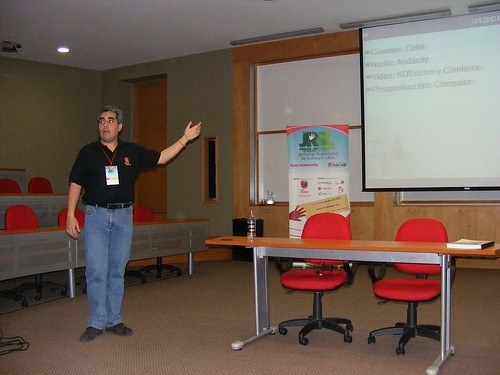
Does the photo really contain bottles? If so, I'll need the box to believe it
[247,210,256,240]
[267,191,275,204]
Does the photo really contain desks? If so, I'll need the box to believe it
[0,168,26,192]
[205,236,500,375]
[0,193,85,229]
[0,218,209,298]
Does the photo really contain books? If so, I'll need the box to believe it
[447,239,494,250]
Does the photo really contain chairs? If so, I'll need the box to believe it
[275,213,359,345]
[28,177,52,193]
[58,208,147,293]
[0,179,21,192]
[6,206,67,300]
[134,207,182,279]
[368,218,456,355]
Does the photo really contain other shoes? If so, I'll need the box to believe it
[79,326,103,342]
[105,323,133,336]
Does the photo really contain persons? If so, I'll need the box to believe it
[65,104,202,344]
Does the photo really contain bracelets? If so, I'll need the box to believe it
[178,138,187,147]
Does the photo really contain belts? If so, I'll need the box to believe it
[87,201,132,209]
